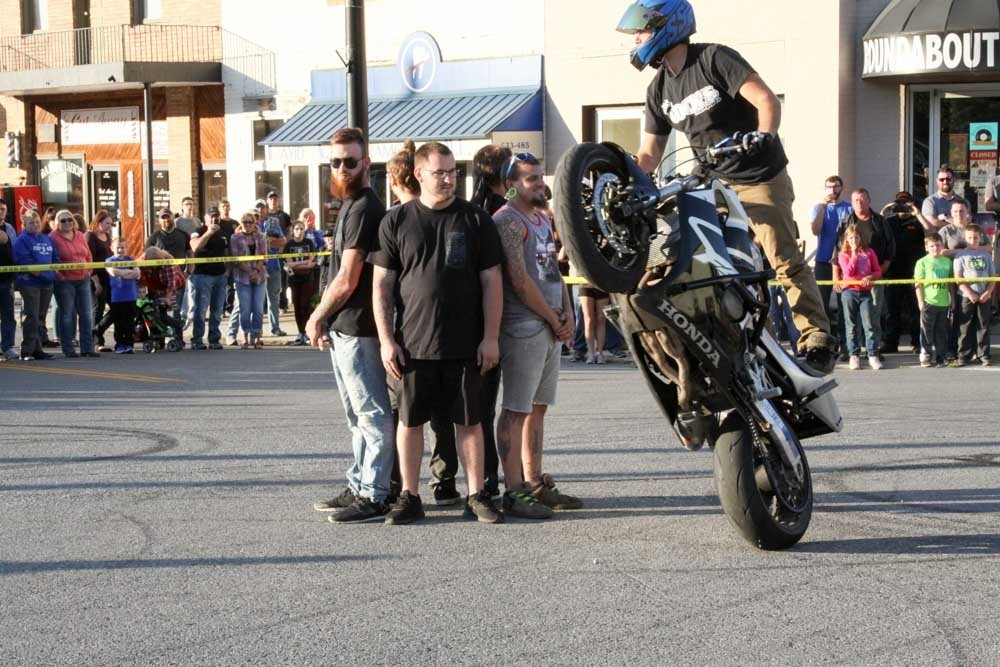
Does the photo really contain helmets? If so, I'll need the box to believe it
[615,0,697,71]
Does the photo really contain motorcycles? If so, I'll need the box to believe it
[552,130,843,551]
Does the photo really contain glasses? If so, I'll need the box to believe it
[60,218,73,223]
[424,168,458,179]
[331,156,365,170]
[242,220,254,225]
[939,176,954,182]
[506,152,535,177]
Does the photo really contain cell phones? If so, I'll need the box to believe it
[214,218,220,225]
[892,204,909,212]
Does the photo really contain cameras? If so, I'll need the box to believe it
[48,207,56,214]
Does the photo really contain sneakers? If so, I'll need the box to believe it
[868,356,883,369]
[313,472,587,525]
[849,356,861,370]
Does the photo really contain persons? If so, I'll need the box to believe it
[302,129,579,527]
[0,191,329,362]
[539,164,999,373]
[614,0,843,375]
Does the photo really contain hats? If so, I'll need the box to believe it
[207,206,220,217]
[255,199,268,208]
[158,207,173,220]
[267,192,280,198]
[250,209,260,216]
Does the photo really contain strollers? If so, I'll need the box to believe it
[131,279,187,354]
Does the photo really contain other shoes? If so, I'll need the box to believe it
[921,355,993,369]
[114,346,126,354]
[125,345,133,354]
[802,347,838,375]
[1,337,101,359]
[191,328,305,350]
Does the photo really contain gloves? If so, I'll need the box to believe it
[734,132,773,155]
